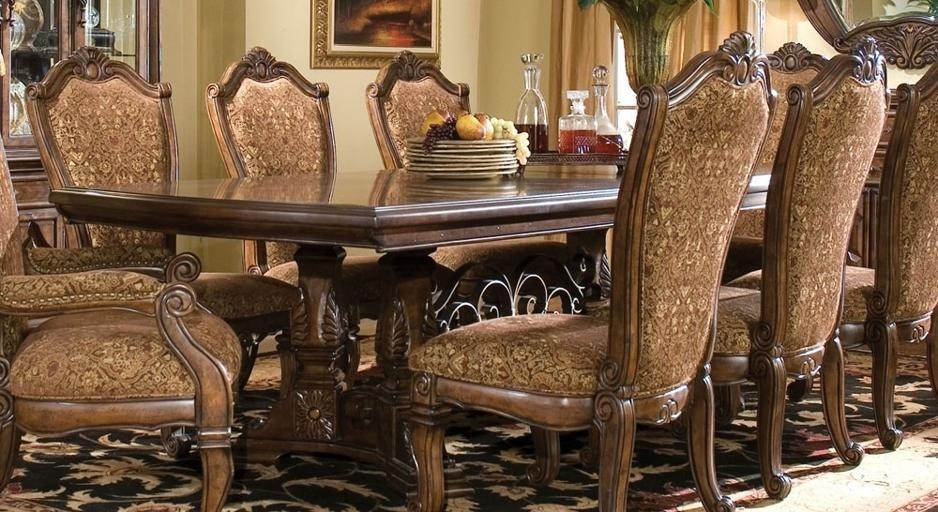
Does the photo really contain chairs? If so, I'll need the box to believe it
[363,51,585,341]
[714,59,938,452]
[577,35,893,498]
[402,29,779,510]
[0,64,242,510]
[719,41,832,284]
[206,47,465,419]
[27,46,304,454]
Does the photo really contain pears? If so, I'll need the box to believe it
[456,115,486,140]
[422,112,445,135]
[474,113,493,139]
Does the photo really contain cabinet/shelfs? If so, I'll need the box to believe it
[846,104,899,270]
[0,0,159,249]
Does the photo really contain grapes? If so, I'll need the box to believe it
[422,118,458,151]
[492,118,530,165]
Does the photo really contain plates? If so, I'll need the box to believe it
[405,133,519,180]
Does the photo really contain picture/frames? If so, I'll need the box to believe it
[309,0,443,69]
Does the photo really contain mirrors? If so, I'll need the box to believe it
[798,0,938,69]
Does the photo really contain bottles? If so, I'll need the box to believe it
[508,52,626,155]
